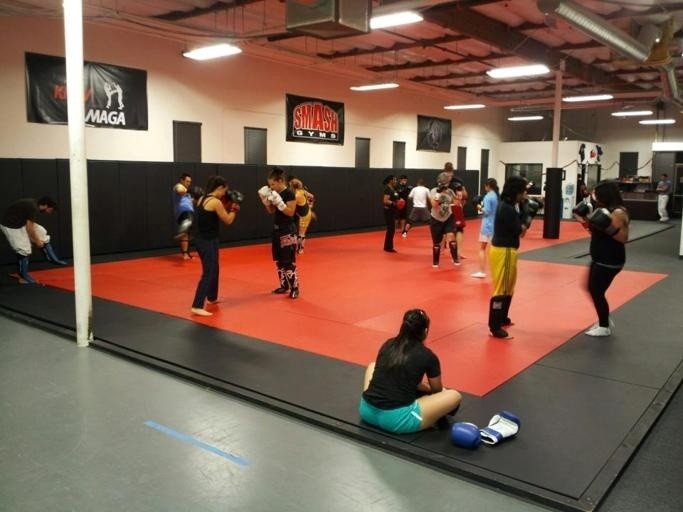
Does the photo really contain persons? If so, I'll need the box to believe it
[0,196,67,285]
[580,184,591,204]
[104,80,125,110]
[359,308,462,434]
[289,179,318,254]
[656,173,672,222]
[191,175,240,316]
[573,180,629,337]
[487,175,543,338]
[382,162,469,267]
[471,178,499,278]
[258,167,299,299]
[171,174,199,260]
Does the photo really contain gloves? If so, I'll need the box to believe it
[192,186,203,200]
[471,195,482,209]
[573,200,593,229]
[588,207,620,238]
[257,185,288,213]
[221,190,245,214]
[518,198,546,229]
[391,198,405,209]
[480,410,521,446]
[450,422,482,451]
